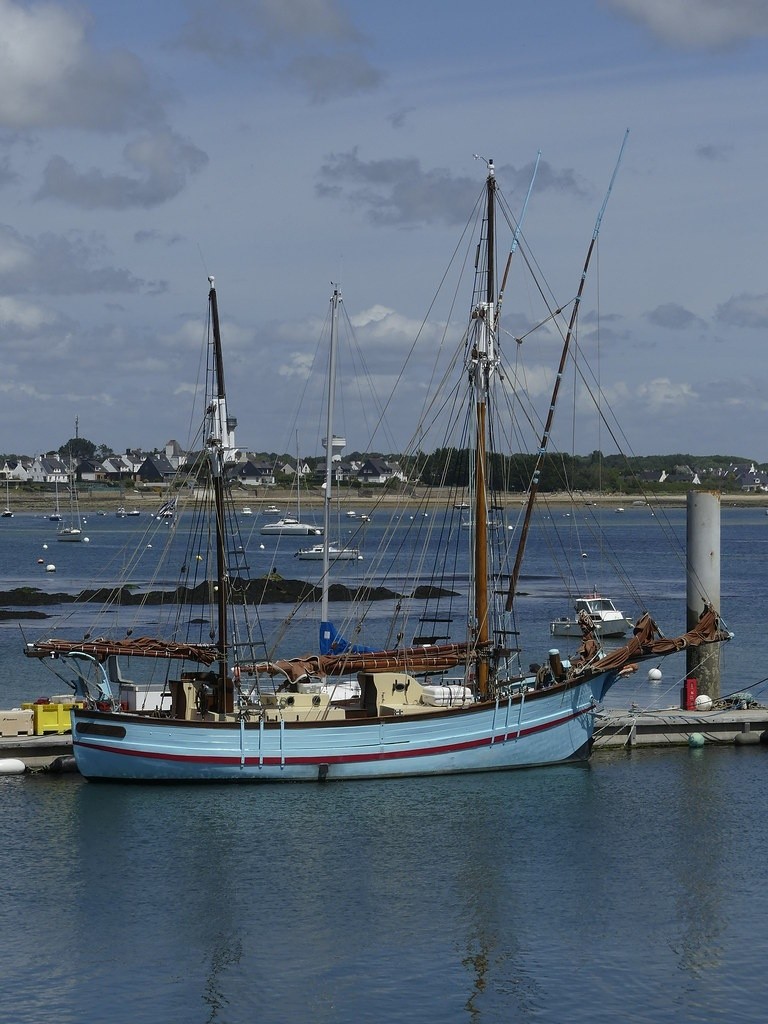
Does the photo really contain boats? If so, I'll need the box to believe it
[300,542,362,562]
[548,597,634,637]
[262,505,281,514]
[261,517,324,536]
[240,507,254,518]
[116,508,141,518]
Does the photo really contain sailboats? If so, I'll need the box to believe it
[55,444,85,541]
[49,475,63,521]
[1,475,14,517]
[51,150,735,782]
